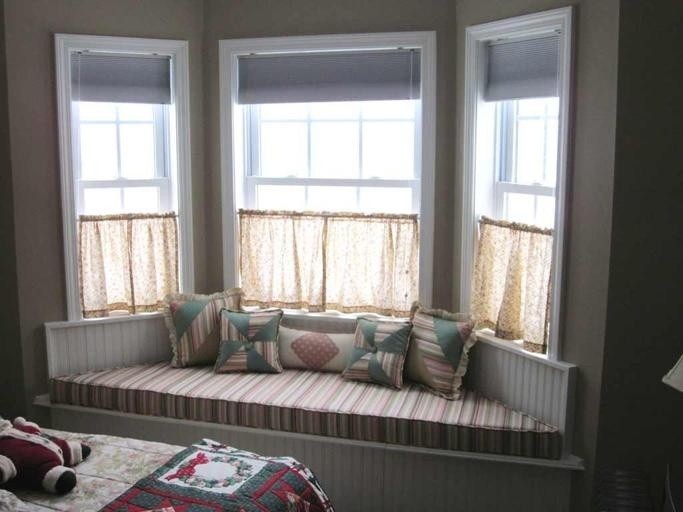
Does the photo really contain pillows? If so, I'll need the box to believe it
[403,301,480,400]
[342,318,414,391]
[278,323,354,372]
[162,288,245,368]
[214,308,284,373]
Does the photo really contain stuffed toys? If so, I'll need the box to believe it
[0,417,91,496]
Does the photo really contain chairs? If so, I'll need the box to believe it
[589,464,677,512]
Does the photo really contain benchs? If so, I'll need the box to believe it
[44,311,578,512]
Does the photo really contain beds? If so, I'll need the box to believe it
[0,417,334,512]
